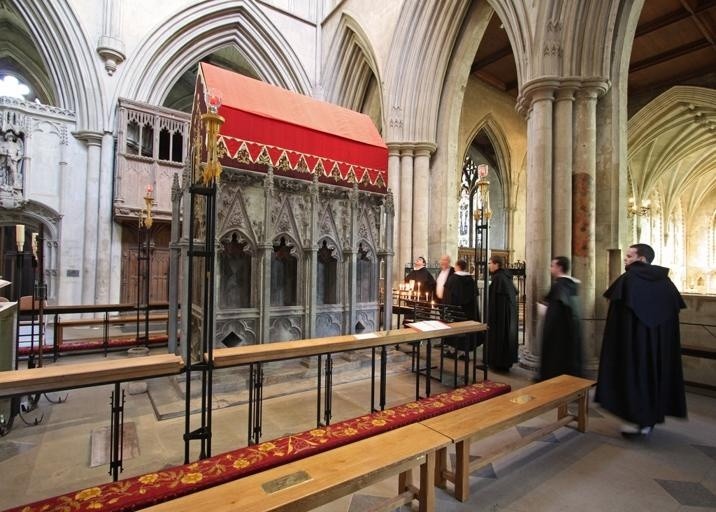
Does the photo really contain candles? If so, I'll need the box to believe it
[16,224,25,243]
[399,281,429,302]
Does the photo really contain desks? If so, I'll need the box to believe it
[18,301,180,364]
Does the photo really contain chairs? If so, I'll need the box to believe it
[19,296,48,347]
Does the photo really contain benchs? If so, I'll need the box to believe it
[419,374,600,498]
[137,424,454,510]
[4,380,515,511]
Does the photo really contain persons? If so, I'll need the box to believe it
[434,252,457,351]
[442,259,485,363]
[403,255,437,348]
[531,254,587,379]
[475,254,519,375]
[593,242,689,437]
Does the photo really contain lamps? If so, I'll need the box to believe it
[627,196,652,218]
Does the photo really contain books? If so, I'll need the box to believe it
[405,317,452,332]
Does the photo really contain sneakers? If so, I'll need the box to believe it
[619,423,655,437]
[434,343,470,362]
